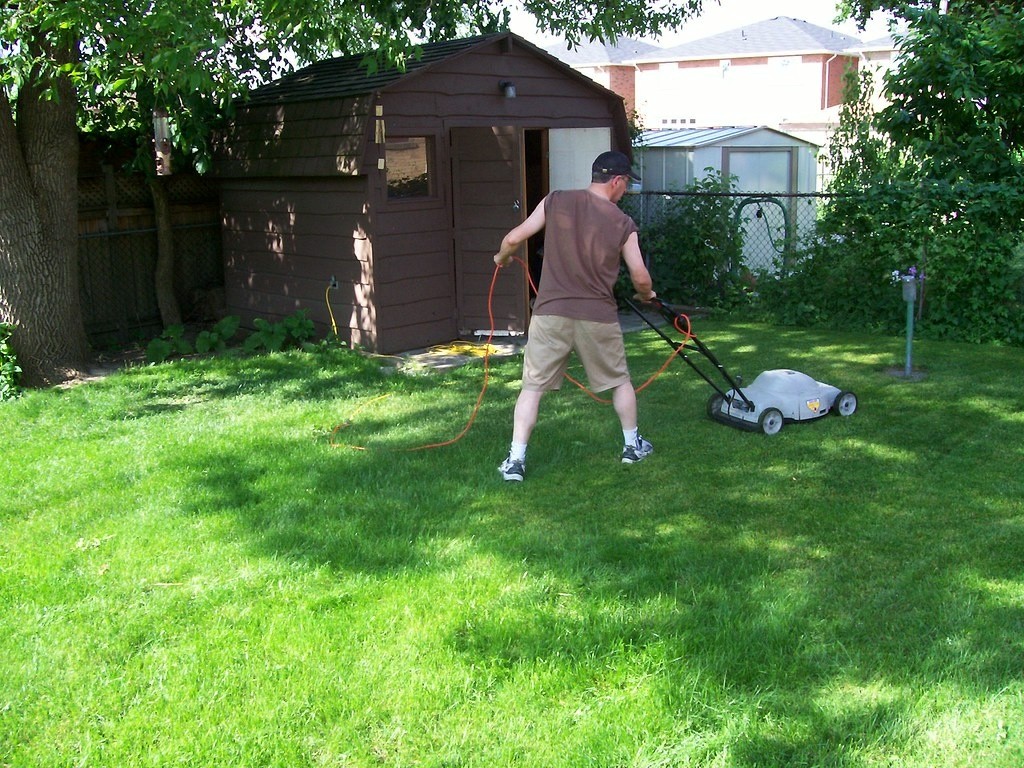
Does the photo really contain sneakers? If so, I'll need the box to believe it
[499,450,526,483]
[622,435,653,464]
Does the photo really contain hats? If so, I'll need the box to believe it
[592,151,641,181]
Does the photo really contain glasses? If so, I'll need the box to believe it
[613,174,630,189]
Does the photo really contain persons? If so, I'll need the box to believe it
[493,151,657,481]
